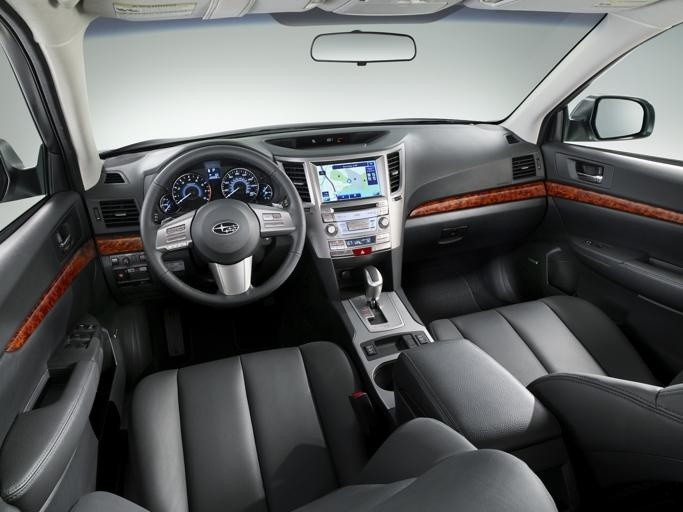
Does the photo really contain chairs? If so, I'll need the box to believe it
[428,295,683,489]
[70,342,559,512]
